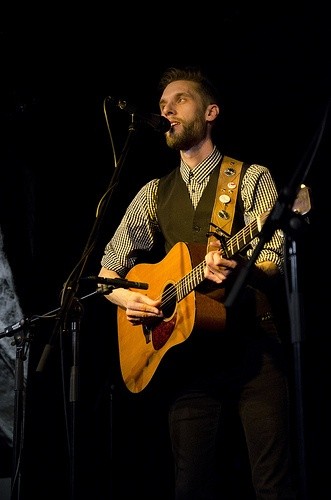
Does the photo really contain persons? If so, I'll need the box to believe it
[96,66,291,500]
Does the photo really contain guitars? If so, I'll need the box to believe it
[117,181,316,396]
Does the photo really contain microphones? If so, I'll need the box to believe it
[107,96,171,132]
[85,275,148,290]
